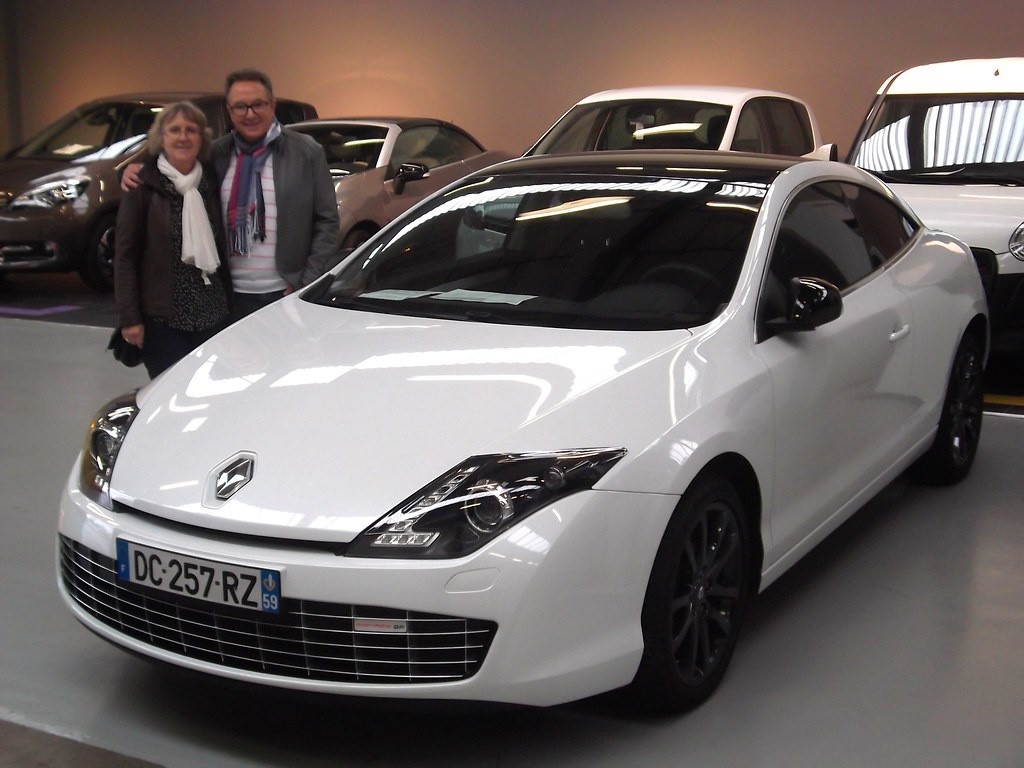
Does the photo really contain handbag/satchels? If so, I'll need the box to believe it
[107,327,144,367]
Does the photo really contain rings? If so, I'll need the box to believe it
[125,337,128,341]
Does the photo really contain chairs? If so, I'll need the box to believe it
[132,113,155,136]
[543,186,651,294]
[328,136,359,163]
[625,104,709,149]
[422,139,452,162]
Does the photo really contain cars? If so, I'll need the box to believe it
[0,91,321,291]
[55,148,992,717]
[284,116,520,254]
[453,85,838,272]
[843,56,1024,276]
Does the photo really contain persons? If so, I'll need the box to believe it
[120,69,341,323]
[115,101,236,383]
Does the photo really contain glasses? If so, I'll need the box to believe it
[162,128,200,137]
[227,100,271,116]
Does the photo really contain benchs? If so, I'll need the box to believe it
[705,116,805,155]
